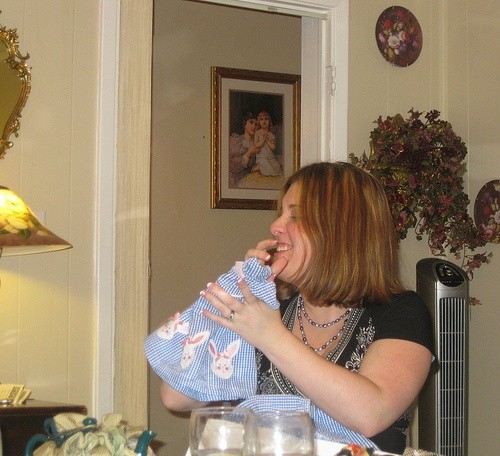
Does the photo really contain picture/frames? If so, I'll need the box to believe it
[210,66,301,212]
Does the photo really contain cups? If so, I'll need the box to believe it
[244,410,316,456]
[189,406,260,456]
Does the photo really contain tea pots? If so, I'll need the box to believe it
[25,417,158,455]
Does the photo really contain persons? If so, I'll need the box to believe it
[160,162,434,456]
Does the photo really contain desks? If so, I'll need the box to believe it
[0,399,88,456]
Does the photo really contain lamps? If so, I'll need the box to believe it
[0,186,73,256]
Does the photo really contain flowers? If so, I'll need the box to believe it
[348,108,498,307]
[473,180,500,243]
[375,6,422,69]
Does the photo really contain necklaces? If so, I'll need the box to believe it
[297,293,352,352]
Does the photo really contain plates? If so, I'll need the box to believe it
[375,6,423,67]
[474,180,500,244]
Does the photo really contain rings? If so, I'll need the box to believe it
[225,309,235,323]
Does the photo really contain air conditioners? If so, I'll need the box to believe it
[416,257,469,456]
